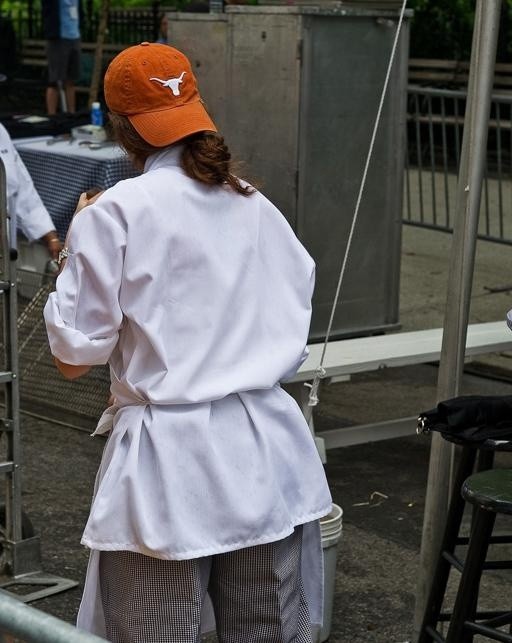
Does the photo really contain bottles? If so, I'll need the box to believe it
[91,102,104,127]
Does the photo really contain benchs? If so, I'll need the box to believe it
[7,36,130,94]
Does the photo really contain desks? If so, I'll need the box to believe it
[10,136,142,248]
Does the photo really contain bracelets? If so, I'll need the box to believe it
[48,237,60,242]
[56,246,68,263]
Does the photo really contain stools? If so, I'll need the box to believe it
[416,424,512,643]
[444,468,512,643]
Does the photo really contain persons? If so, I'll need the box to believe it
[37,0,87,115]
[40,41,334,643]
[1,120,65,265]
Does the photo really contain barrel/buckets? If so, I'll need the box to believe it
[317,502,345,643]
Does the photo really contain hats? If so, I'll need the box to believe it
[104,42,217,147]
